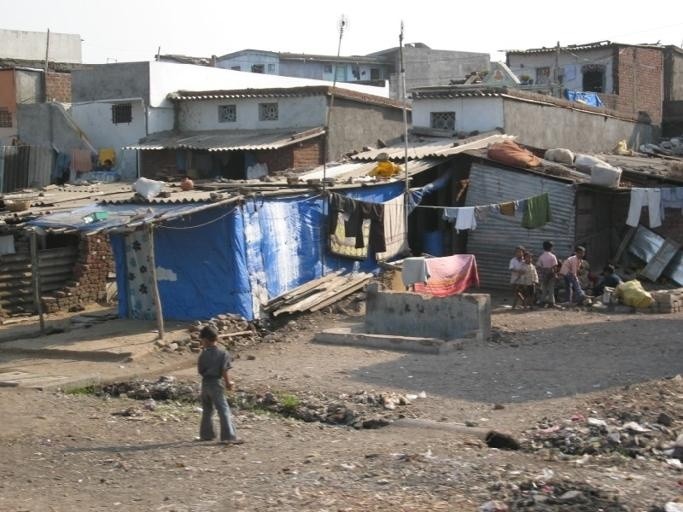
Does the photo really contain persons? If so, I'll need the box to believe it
[511,239,620,308]
[198,327,245,444]
